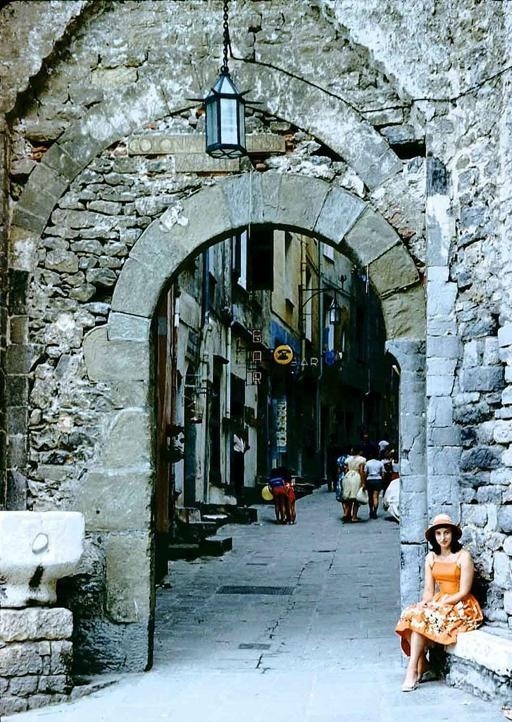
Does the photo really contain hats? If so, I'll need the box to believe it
[425,511,464,541]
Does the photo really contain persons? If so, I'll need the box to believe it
[269,467,298,526]
[394,525,483,692]
[334,448,400,522]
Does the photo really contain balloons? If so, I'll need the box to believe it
[262,485,273,500]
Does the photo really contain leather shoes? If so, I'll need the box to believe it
[400,673,419,692]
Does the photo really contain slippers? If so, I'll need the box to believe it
[341,512,363,522]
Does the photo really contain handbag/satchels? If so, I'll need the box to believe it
[271,486,286,496]
[356,485,369,505]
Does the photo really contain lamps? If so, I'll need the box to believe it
[184,0,262,162]
[298,284,344,328]
